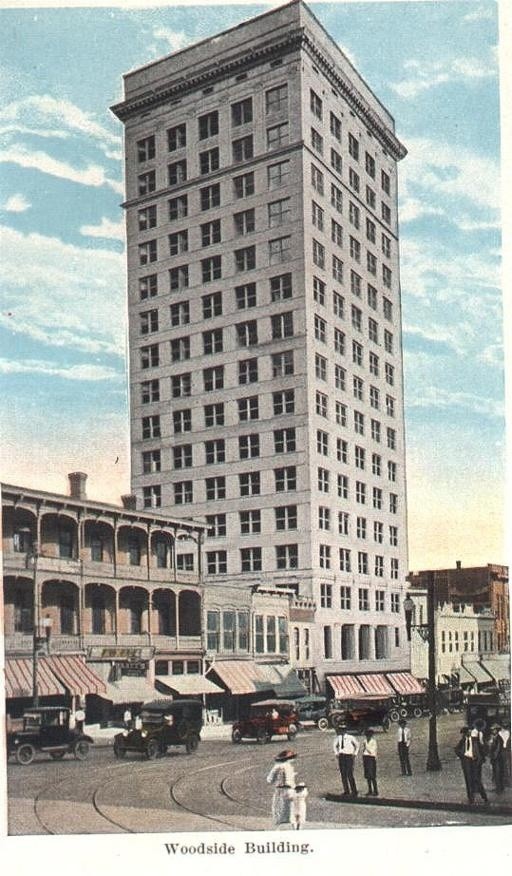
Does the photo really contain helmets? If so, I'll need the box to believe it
[473,718,487,731]
[459,724,472,735]
[490,723,500,731]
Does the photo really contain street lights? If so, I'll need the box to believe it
[29,611,54,708]
[400,570,444,770]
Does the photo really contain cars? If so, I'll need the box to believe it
[113,698,206,759]
[462,677,511,746]
[228,690,436,744]
[7,705,96,765]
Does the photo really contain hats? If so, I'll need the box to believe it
[273,750,298,761]
[294,782,307,789]
[337,723,348,729]
[398,718,407,726]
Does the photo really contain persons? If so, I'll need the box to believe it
[74,707,87,733]
[332,724,360,797]
[265,748,298,828]
[123,707,132,731]
[286,782,310,831]
[451,714,511,809]
[395,718,413,776]
[164,715,174,726]
[360,728,380,797]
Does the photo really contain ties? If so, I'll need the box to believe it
[467,738,470,750]
[402,729,405,741]
[341,736,343,749]
